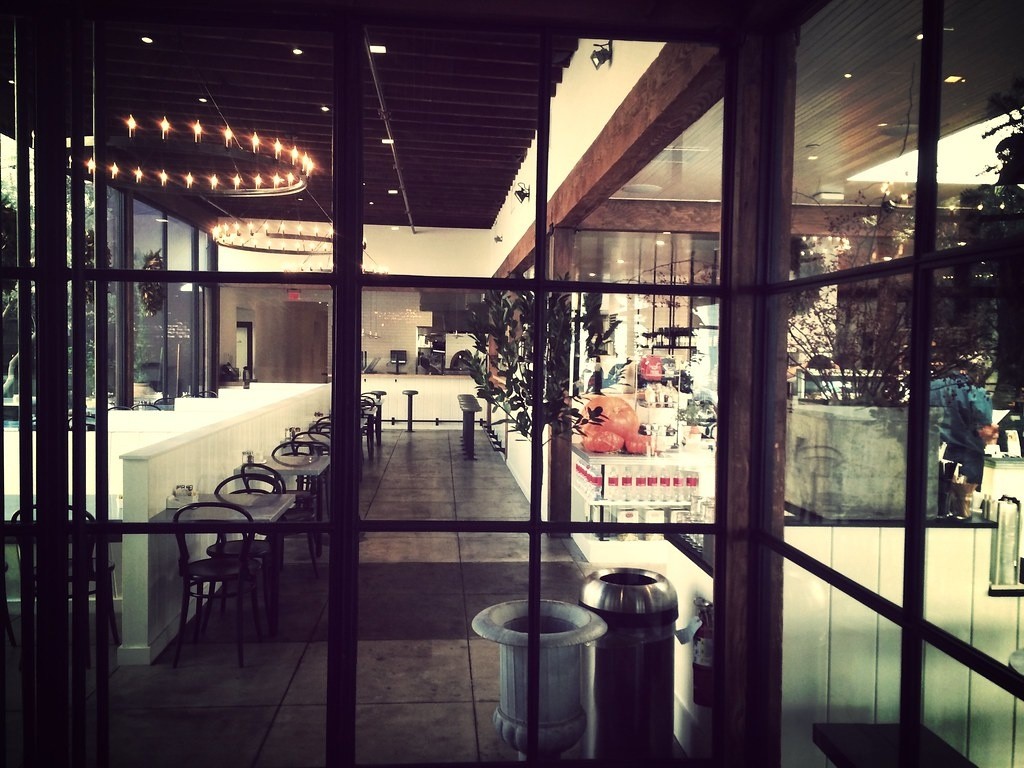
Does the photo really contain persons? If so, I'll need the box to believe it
[929,350,1000,519]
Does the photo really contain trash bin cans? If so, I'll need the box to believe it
[577,568,679,759]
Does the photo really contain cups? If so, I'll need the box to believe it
[677,495,715,552]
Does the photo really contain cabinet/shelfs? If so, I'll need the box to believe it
[632,325,704,450]
[572,444,699,543]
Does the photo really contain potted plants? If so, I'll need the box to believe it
[783,178,1014,518]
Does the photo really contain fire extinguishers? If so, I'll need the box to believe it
[690,595,716,707]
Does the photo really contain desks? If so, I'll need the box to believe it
[282,433,330,457]
[150,492,296,640]
[362,398,386,444]
[311,417,367,430]
[234,457,330,556]
[361,406,378,451]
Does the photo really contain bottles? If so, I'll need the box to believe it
[645,380,678,407]
[243,451,254,464]
[175,485,193,502]
[686,466,698,502]
[672,466,684,502]
[660,467,670,501]
[622,465,632,501]
[312,412,324,422]
[284,427,304,439]
[575,459,603,501]
[648,467,657,501]
[636,466,646,501]
[608,466,619,501]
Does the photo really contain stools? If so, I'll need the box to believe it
[402,391,419,432]
[457,394,483,462]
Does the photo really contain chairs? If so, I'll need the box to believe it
[200,474,286,639]
[173,502,265,668]
[12,504,122,673]
[273,392,381,527]
[240,463,318,577]
[68,392,217,432]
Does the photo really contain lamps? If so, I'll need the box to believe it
[83,12,386,276]
[591,40,613,71]
[514,182,530,203]
[494,235,503,244]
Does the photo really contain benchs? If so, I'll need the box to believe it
[812,719,982,768]
